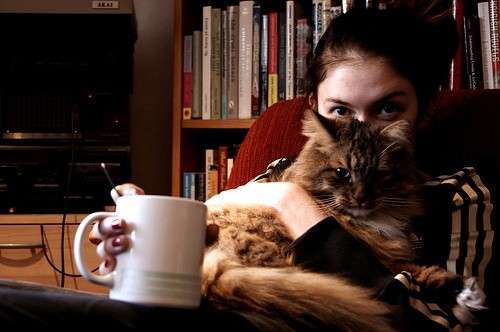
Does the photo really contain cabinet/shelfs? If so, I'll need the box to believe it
[172,0,500,202]
[0,214,110,293]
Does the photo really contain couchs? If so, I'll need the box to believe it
[224,89,500,204]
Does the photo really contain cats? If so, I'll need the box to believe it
[200,108,487,332]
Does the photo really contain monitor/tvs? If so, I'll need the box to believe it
[0,12,133,94]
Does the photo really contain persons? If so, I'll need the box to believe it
[1,7,500,332]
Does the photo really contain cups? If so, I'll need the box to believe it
[73,195,207,308]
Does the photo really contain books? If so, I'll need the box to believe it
[181,0,500,200]
